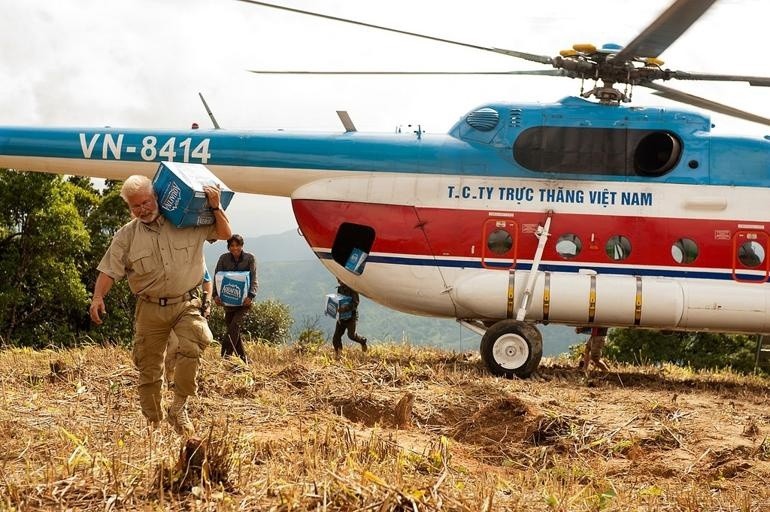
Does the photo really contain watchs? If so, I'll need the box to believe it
[212,203,222,212]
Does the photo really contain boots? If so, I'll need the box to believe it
[165,392,196,436]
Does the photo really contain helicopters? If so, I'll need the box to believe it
[0,0,770,380]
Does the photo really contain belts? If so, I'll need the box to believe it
[142,288,198,306]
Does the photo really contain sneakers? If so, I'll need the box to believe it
[363,341,368,353]
[336,348,344,360]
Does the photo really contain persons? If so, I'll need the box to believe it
[331,273,369,359]
[87,174,235,438]
[210,234,259,366]
[162,264,216,392]
[578,325,611,376]
